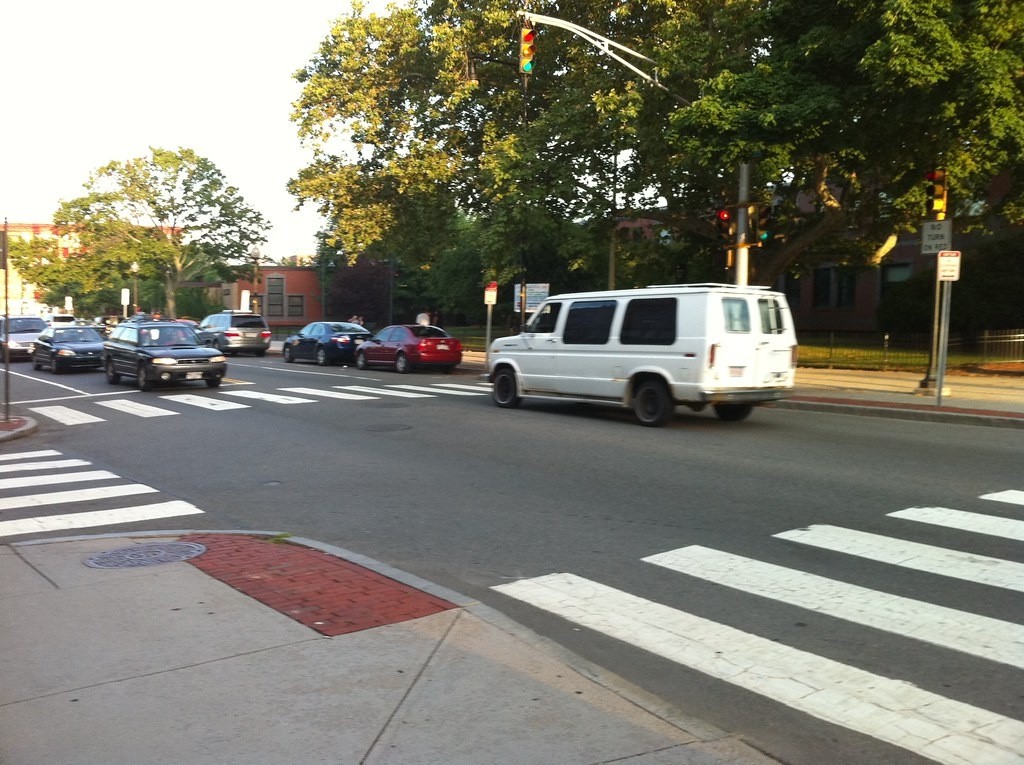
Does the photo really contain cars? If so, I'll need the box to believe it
[283,321,374,366]
[354,324,462,374]
[45,314,199,346]
[0,315,50,362]
[32,324,105,374]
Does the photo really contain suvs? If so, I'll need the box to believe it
[195,309,272,356]
[102,322,228,392]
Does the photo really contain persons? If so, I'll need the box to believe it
[164,331,179,345]
[136,306,146,315]
[416,309,443,330]
[142,333,158,346]
[348,315,365,327]
[79,331,94,340]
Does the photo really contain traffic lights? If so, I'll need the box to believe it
[755,203,775,244]
[925,165,949,213]
[520,26,537,75]
[715,205,729,244]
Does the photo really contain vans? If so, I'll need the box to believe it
[480,282,800,427]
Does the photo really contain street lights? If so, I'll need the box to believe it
[130,261,140,316]
